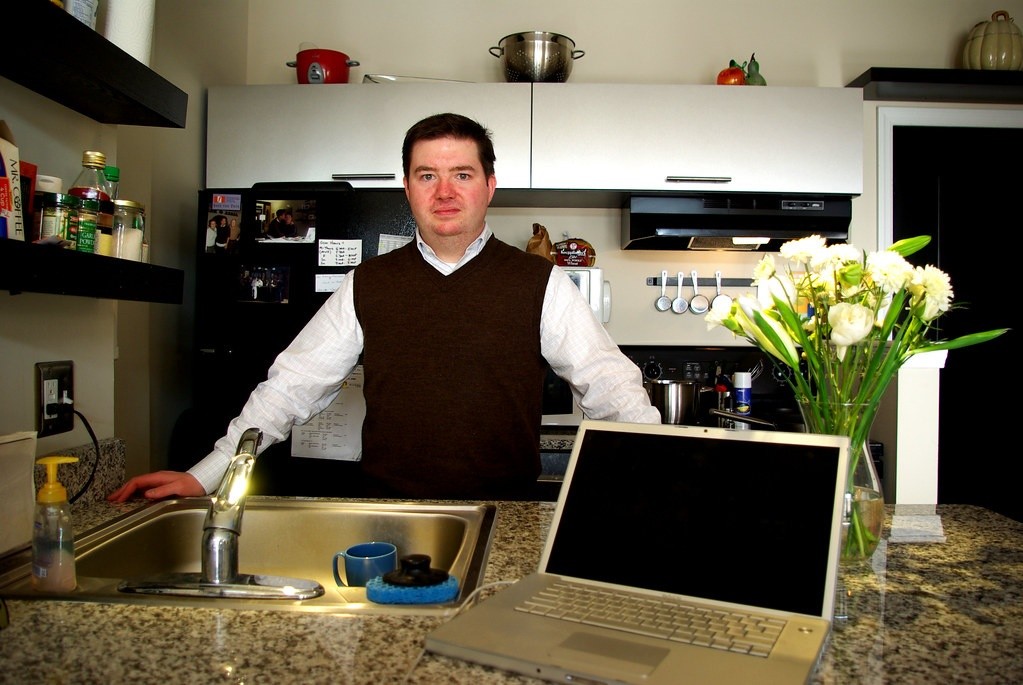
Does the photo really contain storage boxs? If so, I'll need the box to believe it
[0,119,27,241]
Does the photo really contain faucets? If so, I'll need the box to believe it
[200,427,264,584]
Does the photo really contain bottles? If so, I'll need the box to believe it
[734,372,752,415]
[103,165,120,201]
[142,238,148,263]
[68,150,116,257]
[110,198,145,262]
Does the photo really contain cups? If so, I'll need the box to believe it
[332,541,396,586]
[718,391,733,412]
[724,397,733,412]
[836,486,886,567]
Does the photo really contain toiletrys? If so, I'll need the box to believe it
[31,456,80,596]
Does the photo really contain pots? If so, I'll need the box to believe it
[651,380,695,425]
[285,48,360,85]
[488,31,586,84]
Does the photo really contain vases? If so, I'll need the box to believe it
[792,394,885,564]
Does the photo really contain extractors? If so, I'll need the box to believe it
[619,190,853,252]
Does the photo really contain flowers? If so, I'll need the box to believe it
[717,232,1012,562]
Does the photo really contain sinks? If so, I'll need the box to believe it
[0,497,498,617]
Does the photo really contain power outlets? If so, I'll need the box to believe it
[33,361,74,438]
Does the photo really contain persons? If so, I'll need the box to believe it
[206,216,240,252]
[106,114,660,503]
[269,210,296,238]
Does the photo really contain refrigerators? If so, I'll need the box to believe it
[194,183,419,500]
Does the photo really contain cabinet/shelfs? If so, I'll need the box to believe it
[0,0,190,306]
[531,82,864,196]
[204,82,532,190]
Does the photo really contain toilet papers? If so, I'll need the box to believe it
[102,0,156,68]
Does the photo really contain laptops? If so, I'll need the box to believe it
[423,419,852,685]
